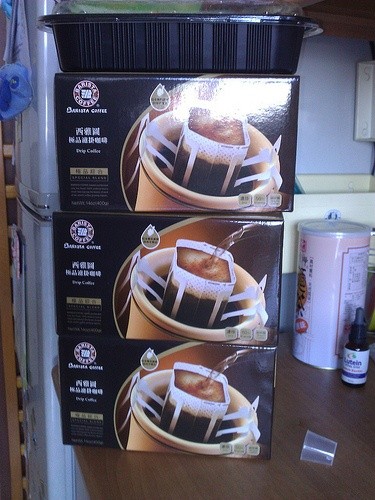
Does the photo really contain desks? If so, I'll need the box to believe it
[50,322,375,500]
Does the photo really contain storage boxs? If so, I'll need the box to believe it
[34,0,326,461]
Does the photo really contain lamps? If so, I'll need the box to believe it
[353,60,375,175]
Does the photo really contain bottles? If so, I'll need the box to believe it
[341,308,370,387]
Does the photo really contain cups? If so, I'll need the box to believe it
[125,238,266,344]
[125,360,258,457]
[134,97,281,214]
[299,429,336,468]
